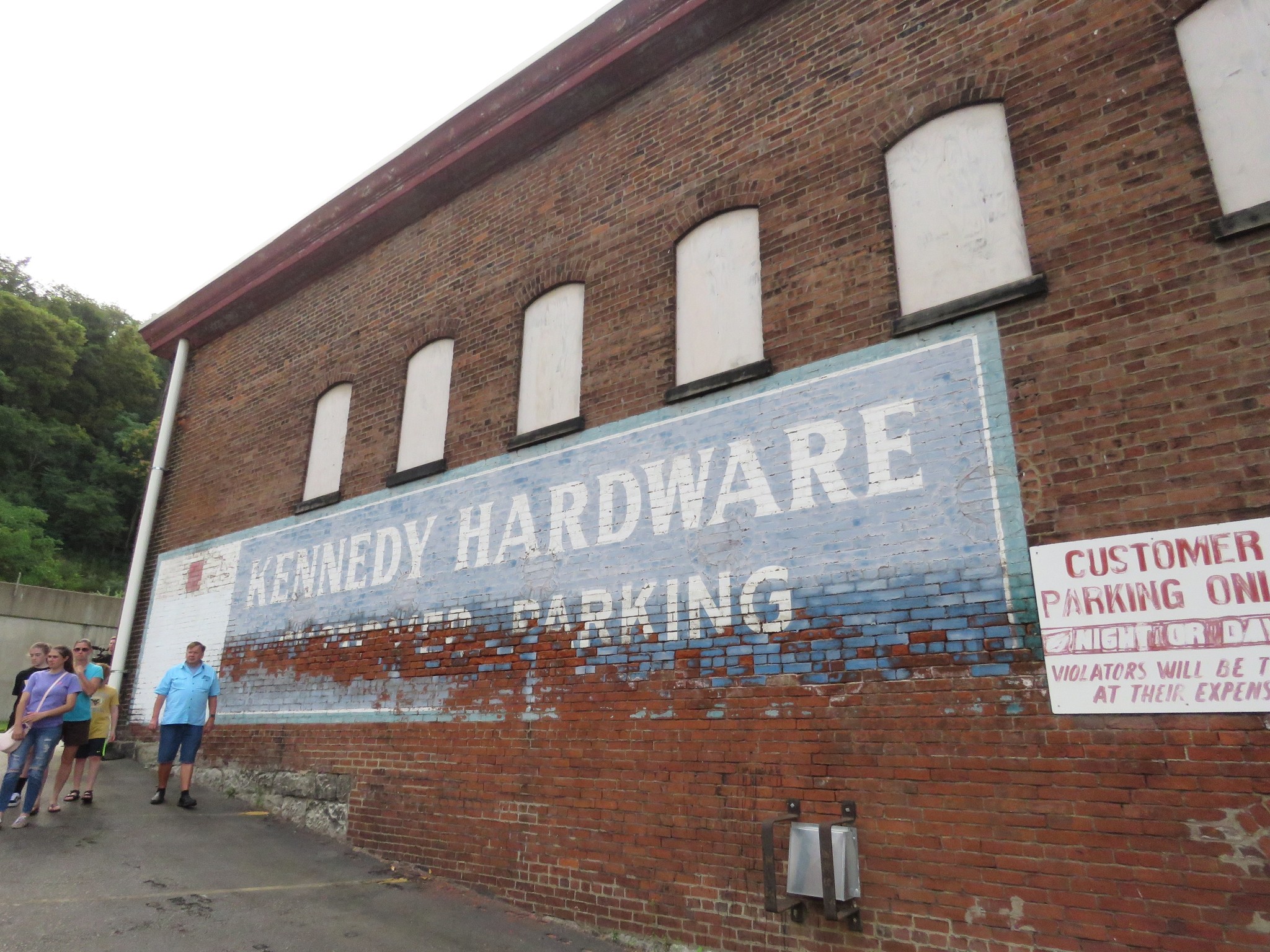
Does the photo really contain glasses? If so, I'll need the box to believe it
[74,648,89,652]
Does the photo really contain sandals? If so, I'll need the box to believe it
[63,790,80,802]
[81,791,93,801]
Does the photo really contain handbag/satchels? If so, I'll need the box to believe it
[0,721,31,753]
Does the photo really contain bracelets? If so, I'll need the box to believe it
[209,714,215,717]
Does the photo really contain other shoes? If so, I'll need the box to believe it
[150,788,166,804]
[178,796,198,807]
[10,816,29,829]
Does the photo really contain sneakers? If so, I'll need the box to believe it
[8,793,22,806]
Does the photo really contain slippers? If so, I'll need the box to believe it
[48,804,61,812]
[30,807,39,815]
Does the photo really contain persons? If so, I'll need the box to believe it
[102,636,117,684]
[148,642,221,807]
[29,639,103,816]
[63,662,119,801]
[0,645,83,828]
[0,642,51,807]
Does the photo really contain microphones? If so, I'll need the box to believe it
[90,645,105,651]
